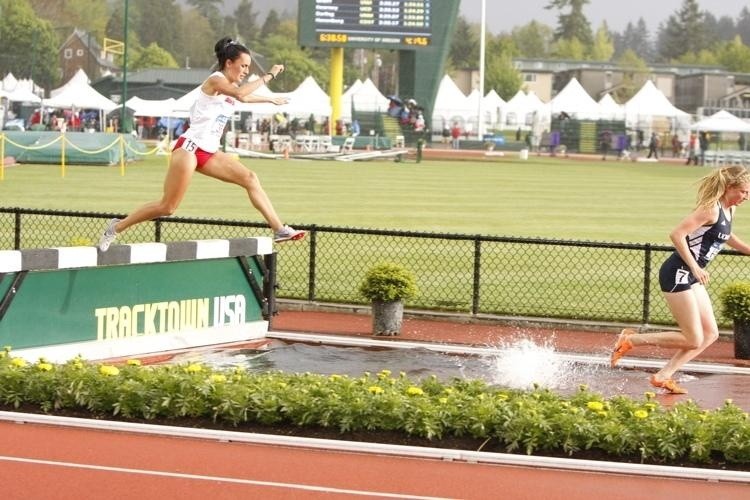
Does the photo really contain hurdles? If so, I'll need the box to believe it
[0,237,279,365]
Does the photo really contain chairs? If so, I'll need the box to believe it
[237,133,355,154]
[705,151,750,167]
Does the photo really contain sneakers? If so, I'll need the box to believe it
[610,328,638,369]
[273,224,306,242]
[98,217,120,253]
[651,374,688,394]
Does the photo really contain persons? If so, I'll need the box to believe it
[243,113,360,136]
[97,33,306,254]
[451,122,461,150]
[385,94,427,132]
[609,162,749,395]
[513,125,709,166]
[0,109,189,141]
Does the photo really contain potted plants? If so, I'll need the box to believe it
[719,280,750,359]
[555,145,566,153]
[356,267,423,337]
[482,142,496,151]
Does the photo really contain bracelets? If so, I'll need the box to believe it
[266,72,275,79]
